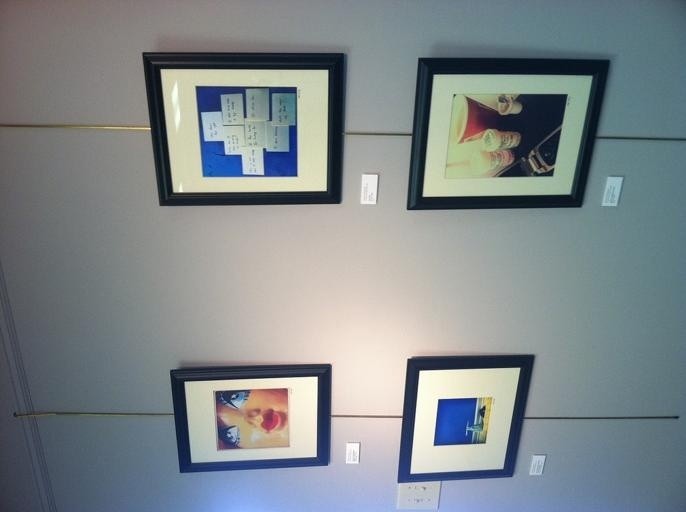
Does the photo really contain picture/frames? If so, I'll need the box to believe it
[396,353,538,484]
[168,363,336,472]
[140,50,347,205]
[404,58,611,210]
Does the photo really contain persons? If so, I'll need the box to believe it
[216,390,289,450]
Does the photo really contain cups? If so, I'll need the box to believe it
[450,95,524,168]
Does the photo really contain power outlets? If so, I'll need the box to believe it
[396,482,442,509]
